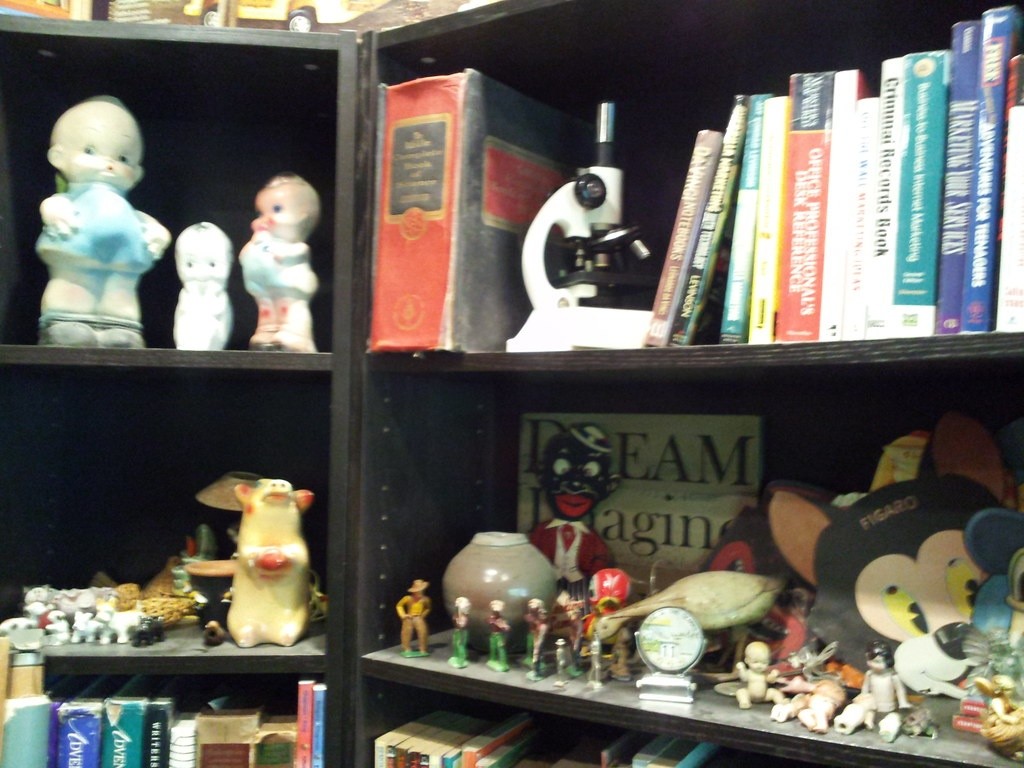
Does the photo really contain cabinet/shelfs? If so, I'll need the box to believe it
[0,14,358,768]
[345,0,1024,768]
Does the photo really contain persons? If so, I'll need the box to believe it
[34,97,319,351]
[449,597,629,687]
[862,640,914,713]
[397,581,432,654]
[735,642,791,710]
[990,675,1024,762]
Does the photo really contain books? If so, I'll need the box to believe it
[0,681,325,768]
[376,709,718,767]
[643,4,1024,348]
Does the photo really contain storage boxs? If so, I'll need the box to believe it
[367,69,595,357]
